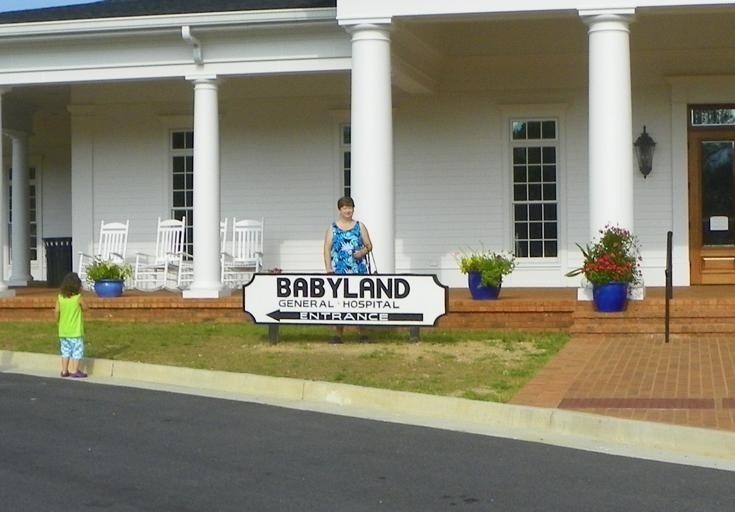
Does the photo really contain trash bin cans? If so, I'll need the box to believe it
[42,237,72,287]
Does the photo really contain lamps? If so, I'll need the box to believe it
[634,125,657,180]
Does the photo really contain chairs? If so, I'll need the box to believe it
[77,219,130,293]
[135,215,263,293]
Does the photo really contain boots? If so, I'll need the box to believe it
[359,336,369,344]
[328,336,344,343]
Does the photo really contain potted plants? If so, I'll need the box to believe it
[452,247,517,300]
[87,262,133,298]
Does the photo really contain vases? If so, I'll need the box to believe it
[592,280,631,312]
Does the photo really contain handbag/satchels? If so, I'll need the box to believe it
[365,245,378,275]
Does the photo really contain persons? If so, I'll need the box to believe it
[54,273,89,378]
[324,197,373,346]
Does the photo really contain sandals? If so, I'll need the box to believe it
[61,370,87,378]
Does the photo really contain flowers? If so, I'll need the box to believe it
[561,226,644,285]
